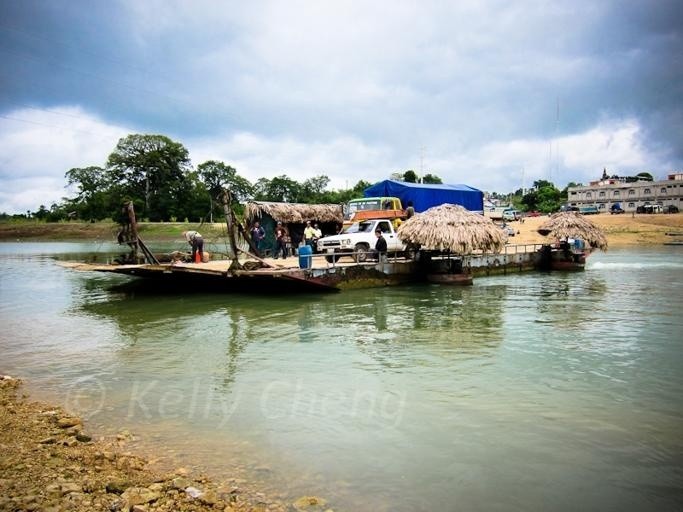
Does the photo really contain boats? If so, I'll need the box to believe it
[424,248,476,286]
[534,243,587,272]
[84,191,596,295]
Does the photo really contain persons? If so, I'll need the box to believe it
[248,220,265,260]
[181,230,204,262]
[313,223,322,254]
[385,202,391,210]
[301,220,320,254]
[403,201,414,219]
[375,228,387,263]
[272,221,288,259]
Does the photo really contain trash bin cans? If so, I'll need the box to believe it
[298,245,312,269]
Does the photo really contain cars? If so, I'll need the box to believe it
[608,207,625,215]
[525,210,541,217]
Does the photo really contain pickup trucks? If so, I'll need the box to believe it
[316,219,442,263]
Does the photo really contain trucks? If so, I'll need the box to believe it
[489,204,524,236]
[340,178,484,235]
[635,203,679,215]
[566,205,600,216]
